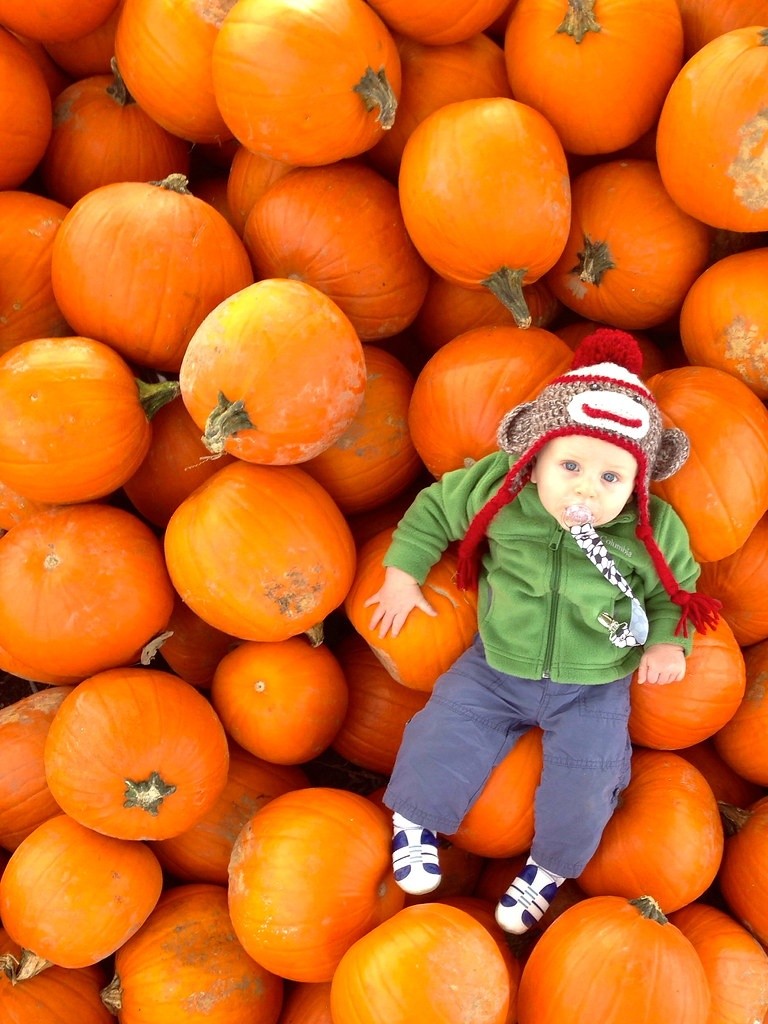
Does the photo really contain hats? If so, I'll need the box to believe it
[455,329,722,637]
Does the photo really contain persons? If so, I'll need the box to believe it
[362,328,722,935]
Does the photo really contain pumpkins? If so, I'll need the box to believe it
[0,0,768,1024]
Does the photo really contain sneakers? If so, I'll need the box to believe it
[393,812,442,896]
[496,855,568,933]
